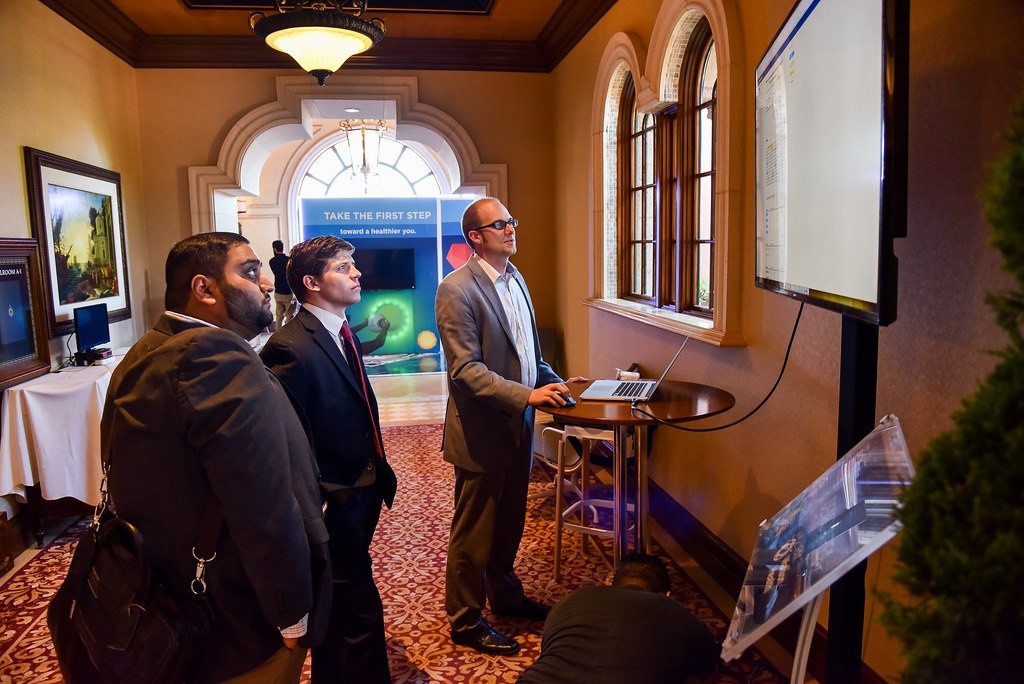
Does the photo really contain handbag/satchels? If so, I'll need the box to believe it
[45,508,213,684]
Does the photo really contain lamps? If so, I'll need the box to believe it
[248,1,386,87]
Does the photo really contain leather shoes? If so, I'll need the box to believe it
[490,597,556,622]
[453,617,521,654]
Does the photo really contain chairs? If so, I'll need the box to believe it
[529,363,642,528]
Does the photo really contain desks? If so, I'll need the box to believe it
[0,348,131,546]
[533,380,735,582]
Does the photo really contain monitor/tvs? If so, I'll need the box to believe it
[73,303,110,353]
[755,0,909,326]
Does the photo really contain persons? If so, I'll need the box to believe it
[269,240,297,332]
[100,232,330,684]
[258,235,397,684]
[515,552,717,684]
[434,198,589,656]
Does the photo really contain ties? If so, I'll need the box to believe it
[338,321,383,459]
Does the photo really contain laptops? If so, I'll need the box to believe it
[579,333,690,401]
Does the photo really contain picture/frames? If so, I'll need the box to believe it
[23,146,131,339]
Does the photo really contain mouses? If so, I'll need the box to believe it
[543,393,576,407]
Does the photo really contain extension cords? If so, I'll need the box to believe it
[95,355,115,365]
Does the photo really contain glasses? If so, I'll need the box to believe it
[471,219,519,230]
